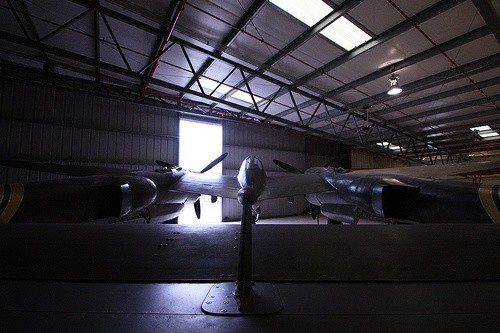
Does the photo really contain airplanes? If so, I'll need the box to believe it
[169,153,332,206]
[271,159,500,224]
[0,152,229,223]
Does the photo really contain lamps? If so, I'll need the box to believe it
[387,73,402,96]
